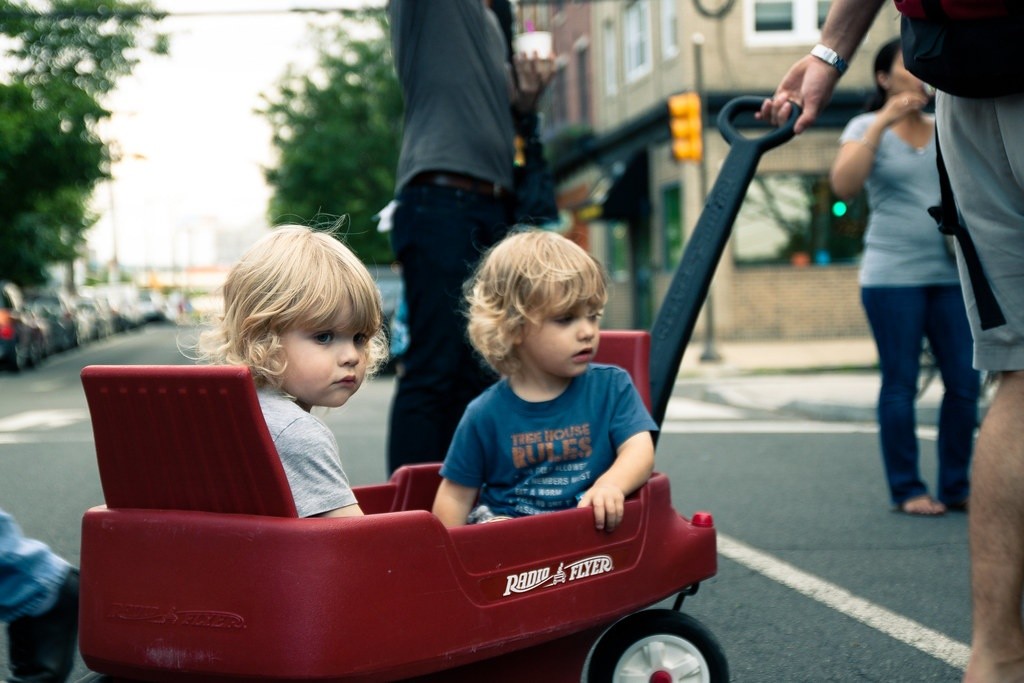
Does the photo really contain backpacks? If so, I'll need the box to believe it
[895,0,1024,104]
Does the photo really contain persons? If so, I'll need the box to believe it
[751,0,1024,682]
[432,223,662,532]
[828,38,982,514]
[384,0,557,482]
[175,207,382,519]
[0,509,81,683]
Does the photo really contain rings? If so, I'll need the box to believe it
[904,99,908,104]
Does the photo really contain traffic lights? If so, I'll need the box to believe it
[664,88,705,164]
[828,197,851,224]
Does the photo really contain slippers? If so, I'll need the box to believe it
[894,494,944,516]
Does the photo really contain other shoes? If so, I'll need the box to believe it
[7,567,81,683]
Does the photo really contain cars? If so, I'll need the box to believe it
[0,276,162,374]
[365,263,411,380]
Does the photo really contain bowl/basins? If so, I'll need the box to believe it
[512,31,553,60]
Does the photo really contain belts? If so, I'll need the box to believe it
[411,171,504,198]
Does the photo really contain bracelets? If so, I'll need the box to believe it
[807,45,849,75]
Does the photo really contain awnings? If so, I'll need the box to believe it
[576,145,650,221]
